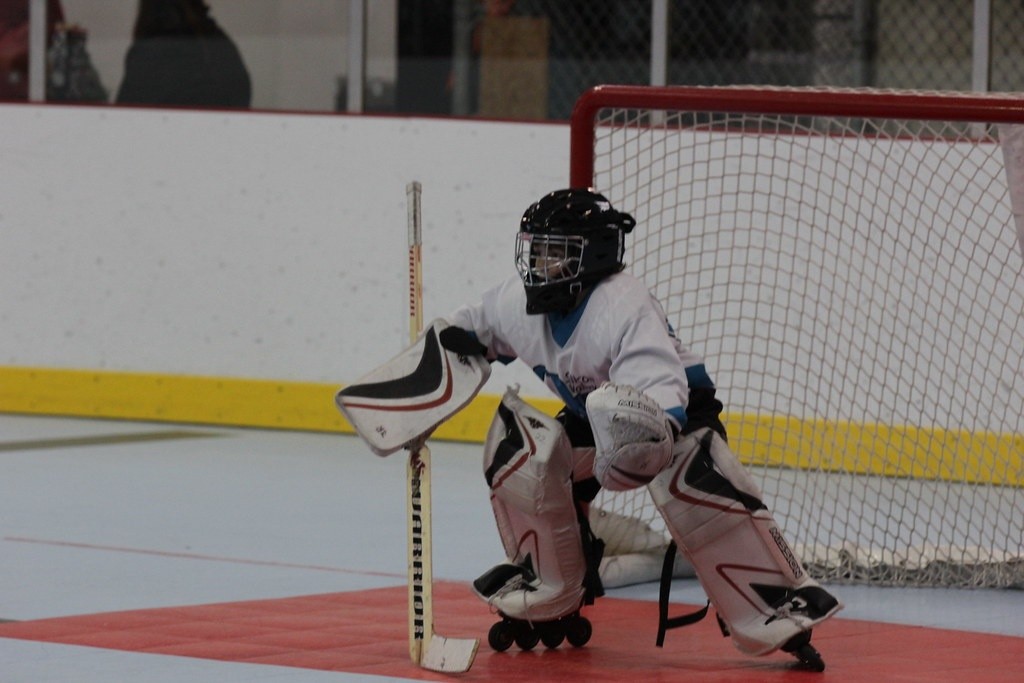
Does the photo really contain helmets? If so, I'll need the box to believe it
[515,185,636,315]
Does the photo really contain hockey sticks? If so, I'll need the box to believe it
[404,180,482,675]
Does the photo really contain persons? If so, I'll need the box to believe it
[334,187,847,671]
[0,0,110,106]
[114,0,254,110]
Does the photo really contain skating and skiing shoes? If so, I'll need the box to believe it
[488,586,593,651]
[781,625,827,673]
